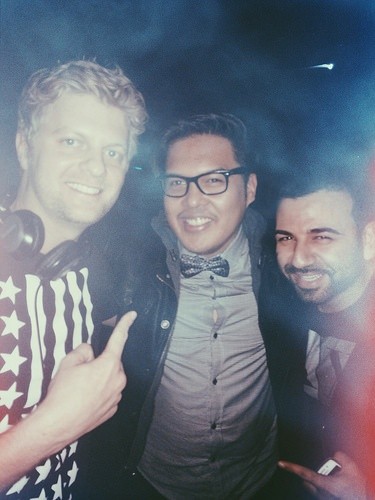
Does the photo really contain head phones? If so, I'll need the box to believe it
[0,209,82,286]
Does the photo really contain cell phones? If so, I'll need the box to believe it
[315,456,342,476]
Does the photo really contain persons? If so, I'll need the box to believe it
[104,111,311,500]
[0,58,149,500]
[275,163,375,500]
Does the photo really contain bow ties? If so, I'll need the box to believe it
[179,254,230,278]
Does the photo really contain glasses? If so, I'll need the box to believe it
[157,167,246,198]
